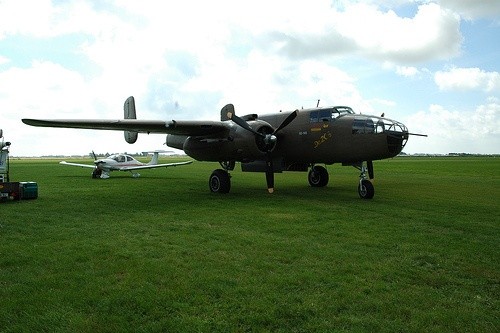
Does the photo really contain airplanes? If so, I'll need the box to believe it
[21,96,429,199]
[58,150,194,179]
